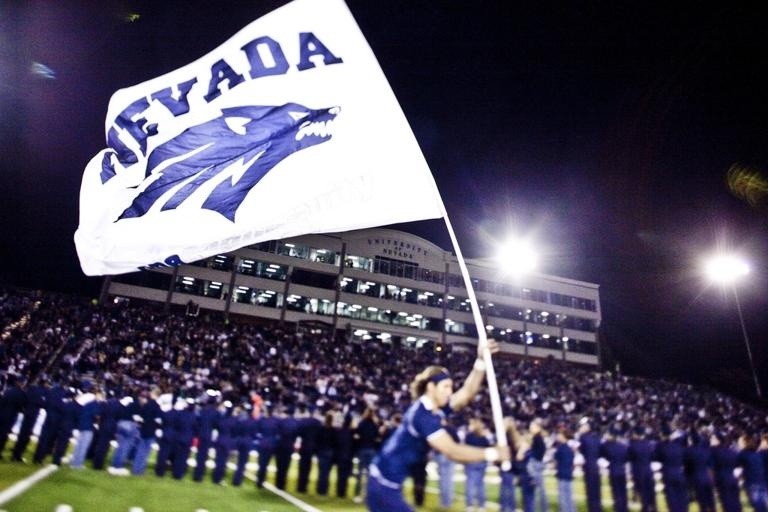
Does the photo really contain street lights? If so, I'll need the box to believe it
[495,234,540,368]
[705,252,764,406]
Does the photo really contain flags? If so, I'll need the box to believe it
[74,0,447,277]
